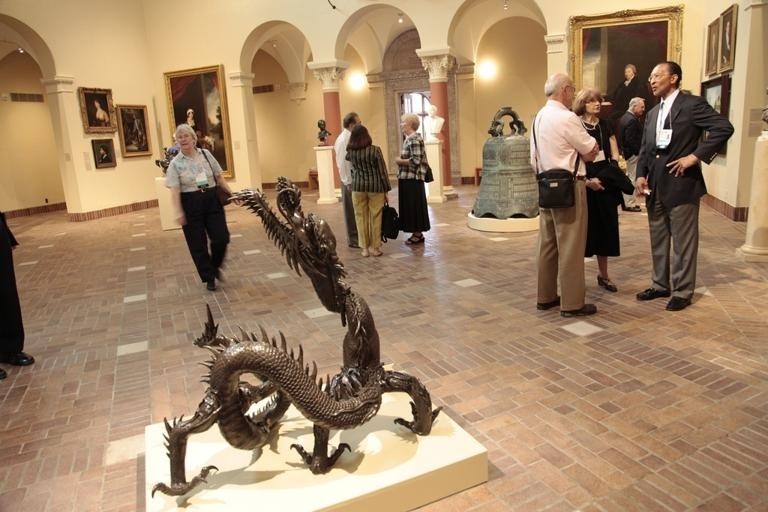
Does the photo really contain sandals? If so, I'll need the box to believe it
[597,275,617,293]
[406,234,425,245]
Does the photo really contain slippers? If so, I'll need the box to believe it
[360,250,370,257]
[374,251,383,256]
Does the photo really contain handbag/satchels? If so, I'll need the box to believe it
[533,112,581,208]
[201,149,230,206]
[381,199,399,243]
[424,164,433,183]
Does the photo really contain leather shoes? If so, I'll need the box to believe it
[625,207,641,212]
[206,280,215,291]
[214,268,225,282]
[665,296,692,311]
[349,243,360,248]
[0,368,6,380]
[635,206,640,208]
[636,287,671,301]
[560,304,596,317]
[2,350,34,366]
[537,295,560,310]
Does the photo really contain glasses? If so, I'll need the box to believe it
[648,73,672,82]
[567,86,575,92]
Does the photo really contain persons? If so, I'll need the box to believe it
[93,99,110,124]
[634,61,735,310]
[573,88,634,293]
[396,110,434,247]
[335,111,362,249]
[186,108,196,125]
[617,96,647,215]
[166,125,239,291]
[97,145,112,164]
[1,211,35,383]
[614,62,649,107]
[528,73,600,317]
[345,125,391,257]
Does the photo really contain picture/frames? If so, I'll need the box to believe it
[570,3,684,123]
[78,87,118,134]
[164,63,235,180]
[115,103,152,157]
[700,74,730,156]
[91,138,117,169]
[718,4,739,72]
[703,18,721,77]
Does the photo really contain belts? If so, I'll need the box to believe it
[180,187,216,195]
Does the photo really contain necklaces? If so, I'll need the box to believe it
[581,118,600,124]
